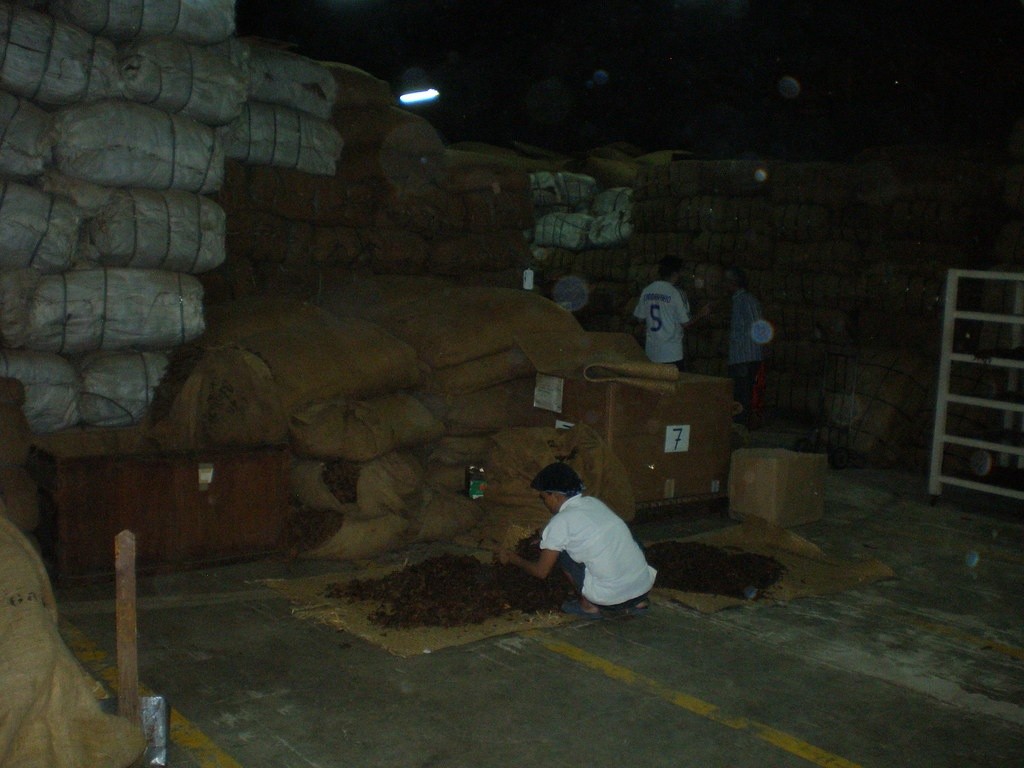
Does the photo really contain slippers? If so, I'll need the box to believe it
[560,599,603,619]
[633,605,649,612]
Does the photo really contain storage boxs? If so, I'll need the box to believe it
[29,440,287,584]
[552,370,735,510]
[727,449,825,530]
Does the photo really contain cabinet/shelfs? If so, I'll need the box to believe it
[926,269,1023,508]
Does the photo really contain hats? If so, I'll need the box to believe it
[530,462,586,496]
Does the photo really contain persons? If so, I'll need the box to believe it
[631,257,712,372]
[724,266,770,432]
[498,464,658,620]
